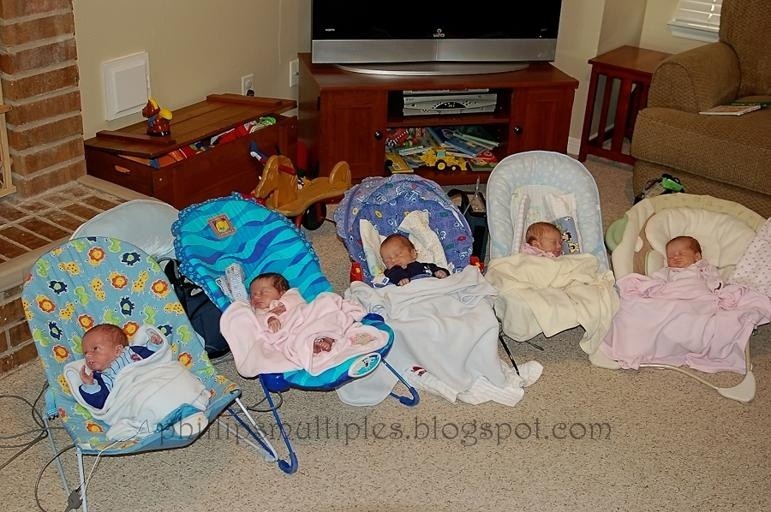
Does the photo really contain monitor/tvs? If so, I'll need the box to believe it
[311,0,562,75]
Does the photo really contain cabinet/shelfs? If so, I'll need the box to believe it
[298,52,582,205]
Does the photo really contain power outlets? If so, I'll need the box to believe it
[241,71,258,97]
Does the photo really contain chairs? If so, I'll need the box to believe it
[629,1,770,219]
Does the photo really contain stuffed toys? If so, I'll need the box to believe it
[633,174,684,205]
[142,96,173,136]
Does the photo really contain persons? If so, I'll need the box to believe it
[520,222,561,258]
[380,234,450,286]
[78,324,163,409]
[652,236,718,282]
[249,273,333,353]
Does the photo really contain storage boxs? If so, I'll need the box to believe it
[79,86,299,212]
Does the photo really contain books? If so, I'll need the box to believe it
[731,96,771,108]
[699,105,761,116]
[385,127,498,174]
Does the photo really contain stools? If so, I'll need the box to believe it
[574,43,675,171]
